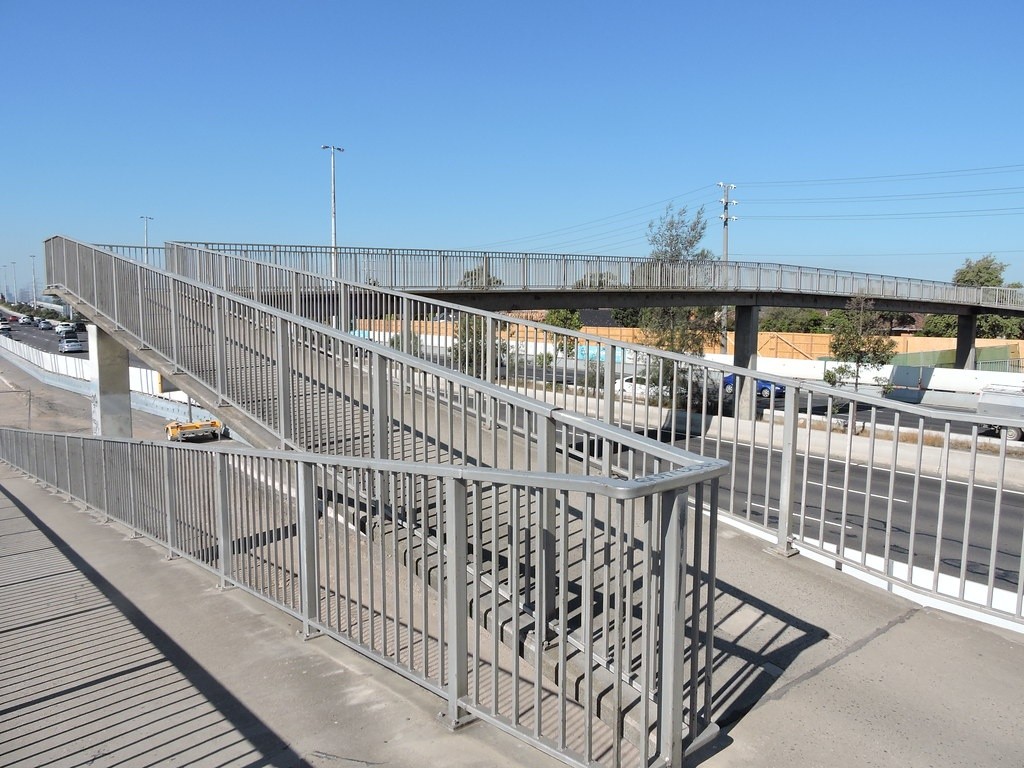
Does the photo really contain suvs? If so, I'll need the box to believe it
[20,316,31,324]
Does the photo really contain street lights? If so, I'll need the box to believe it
[3,265,7,303]
[30,255,36,310]
[139,215,155,265]
[320,144,345,288]
[11,261,18,305]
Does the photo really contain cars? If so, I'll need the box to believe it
[58,338,83,353]
[9,316,18,322]
[60,330,77,339]
[724,373,785,399]
[615,375,687,400]
[0,313,12,340]
[55,323,73,334]
[38,321,52,330]
[18,300,43,309]
[34,318,45,327]
[27,315,34,321]
[72,323,86,332]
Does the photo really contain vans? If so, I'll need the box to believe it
[978,385,1023,442]
